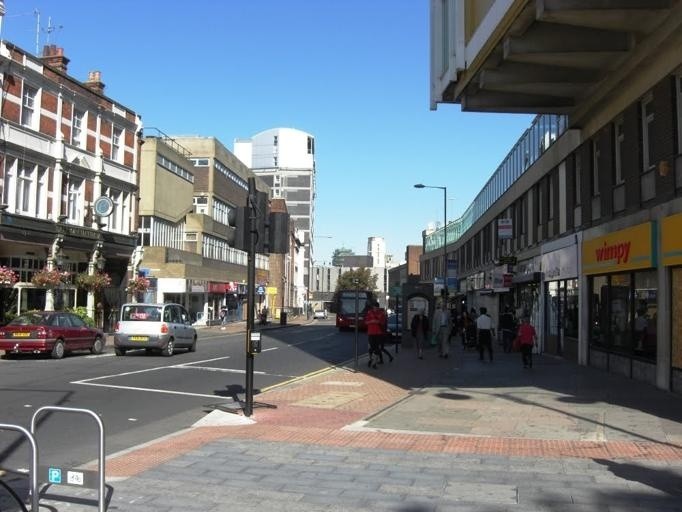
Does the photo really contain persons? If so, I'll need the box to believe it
[323,308,327,320]
[257,306,268,326]
[220,306,228,325]
[365,302,538,369]
[630,300,648,331]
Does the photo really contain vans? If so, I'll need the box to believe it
[114,302,197,356]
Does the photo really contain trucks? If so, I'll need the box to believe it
[336,291,372,331]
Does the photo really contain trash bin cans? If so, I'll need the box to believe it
[280,312,287,325]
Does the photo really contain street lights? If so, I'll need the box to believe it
[413,184,447,291]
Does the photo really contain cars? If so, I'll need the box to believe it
[0,310,106,359]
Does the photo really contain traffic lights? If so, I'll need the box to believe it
[226,206,250,252]
